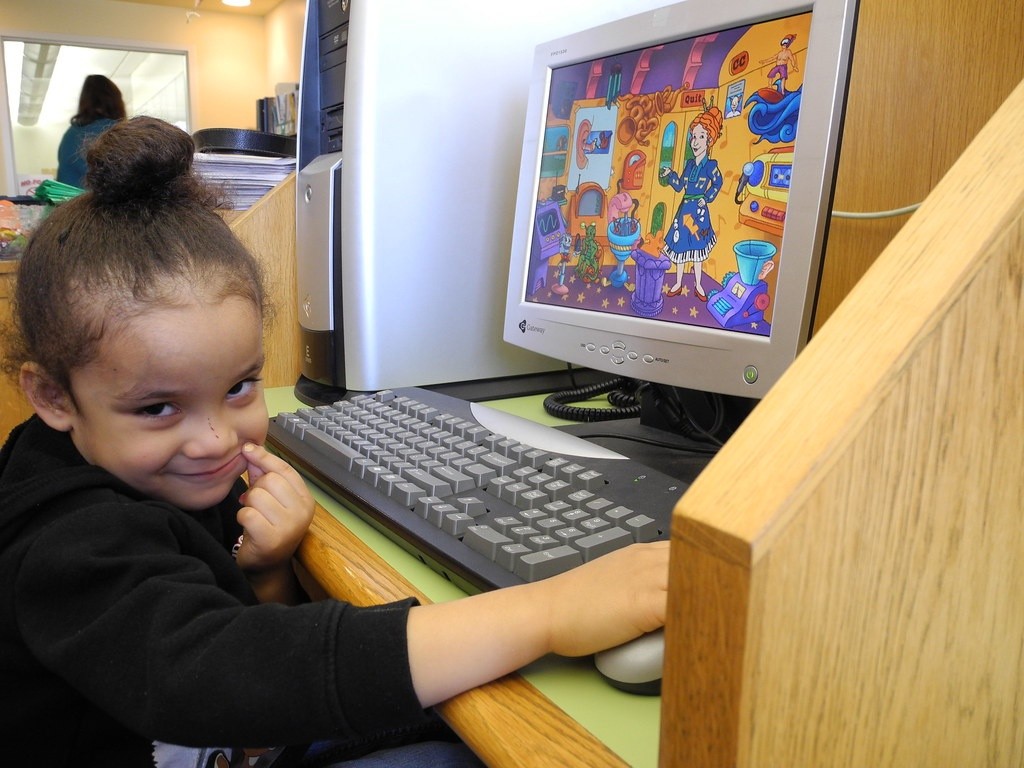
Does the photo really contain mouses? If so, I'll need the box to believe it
[594,627,665,684]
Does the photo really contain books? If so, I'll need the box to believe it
[256,93,298,136]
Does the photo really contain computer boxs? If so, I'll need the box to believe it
[290,0,687,409]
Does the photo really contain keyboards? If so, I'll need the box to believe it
[264,387,692,596]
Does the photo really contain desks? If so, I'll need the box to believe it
[239,345,663,768]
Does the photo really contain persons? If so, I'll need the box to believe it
[56,75,128,189]
[0,117,672,768]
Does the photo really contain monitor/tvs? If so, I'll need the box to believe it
[504,0,861,488]
